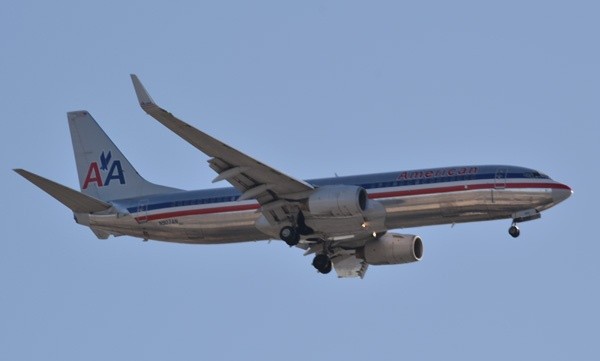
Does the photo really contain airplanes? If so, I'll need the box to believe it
[9,71,573,279]
